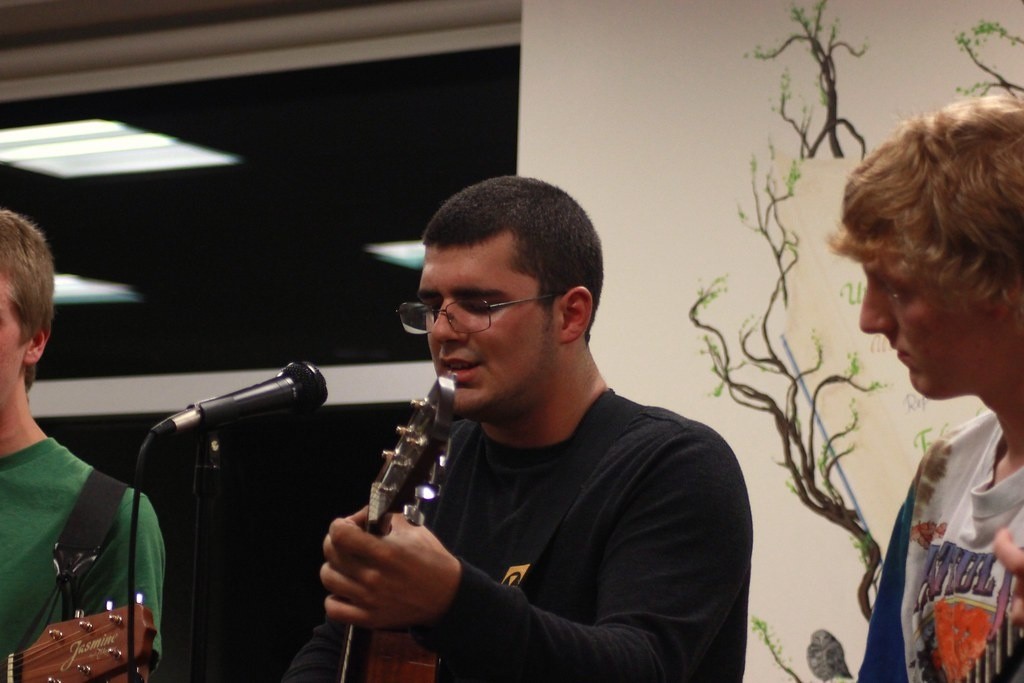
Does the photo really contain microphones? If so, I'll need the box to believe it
[150,362,329,439]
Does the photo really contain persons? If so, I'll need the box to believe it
[0,208,162,681]
[828,101,1024,683]
[287,175,754,683]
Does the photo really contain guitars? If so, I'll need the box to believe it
[0,593,158,683]
[335,373,457,683]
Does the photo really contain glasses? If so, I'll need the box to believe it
[393,294,558,335]
